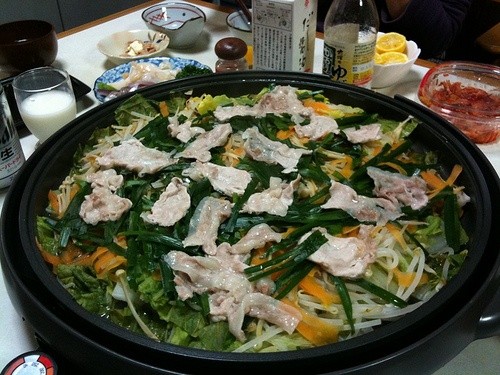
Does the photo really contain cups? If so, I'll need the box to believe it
[12,67,77,145]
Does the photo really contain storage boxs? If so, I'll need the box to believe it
[251,0,318,74]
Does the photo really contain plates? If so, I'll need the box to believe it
[94,57,212,104]
[2,73,91,126]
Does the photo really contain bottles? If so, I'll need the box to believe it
[215,37,250,73]
[322,0,380,90]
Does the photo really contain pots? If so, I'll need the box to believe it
[0,69,500,375]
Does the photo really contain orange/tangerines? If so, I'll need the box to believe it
[374,32,409,64]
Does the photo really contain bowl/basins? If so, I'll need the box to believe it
[98,29,170,66]
[226,9,254,45]
[0,19,58,73]
[142,2,206,49]
[417,61,500,144]
[358,31,421,88]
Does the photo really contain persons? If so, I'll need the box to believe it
[316,0,473,59]
[470,0,500,53]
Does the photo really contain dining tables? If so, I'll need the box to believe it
[0,0,500,375]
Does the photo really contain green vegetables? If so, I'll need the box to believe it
[32,85,471,356]
[97,63,211,92]
